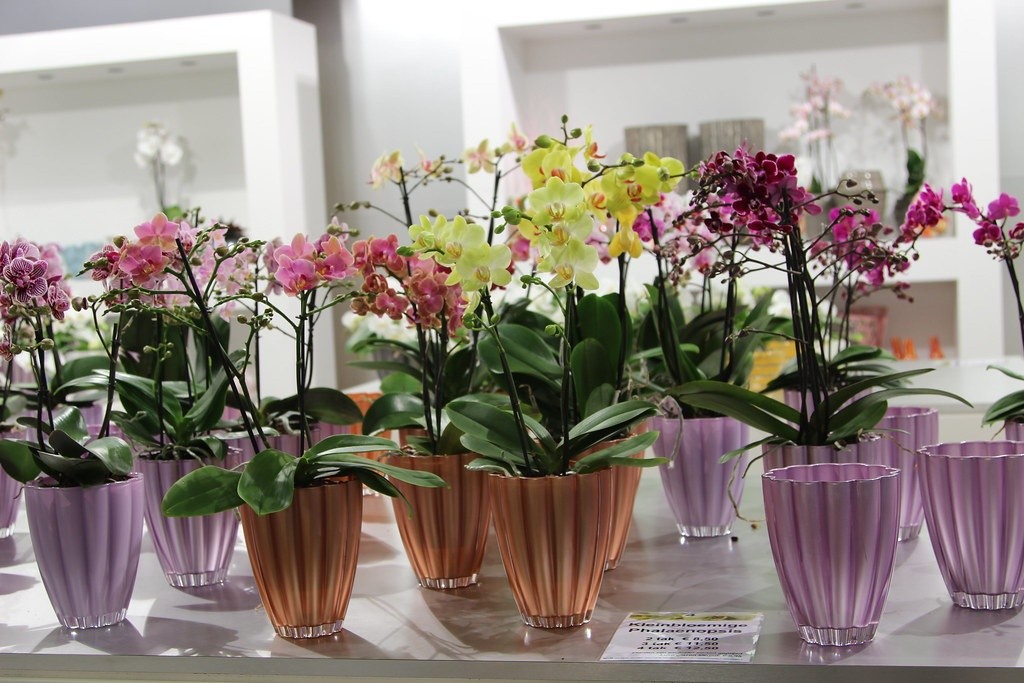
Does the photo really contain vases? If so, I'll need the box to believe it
[783,387,872,430]
[387,451,492,590]
[915,439,1024,609]
[647,416,748,537]
[835,170,886,217]
[1005,422,1024,441]
[760,440,890,467]
[761,460,901,645]
[834,405,939,541]
[490,472,614,628]
[0,391,363,639]
[575,432,646,570]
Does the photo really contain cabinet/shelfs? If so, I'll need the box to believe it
[0,10,336,401]
[464,0,1006,362]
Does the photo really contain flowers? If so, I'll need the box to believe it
[0,64,1024,475]
